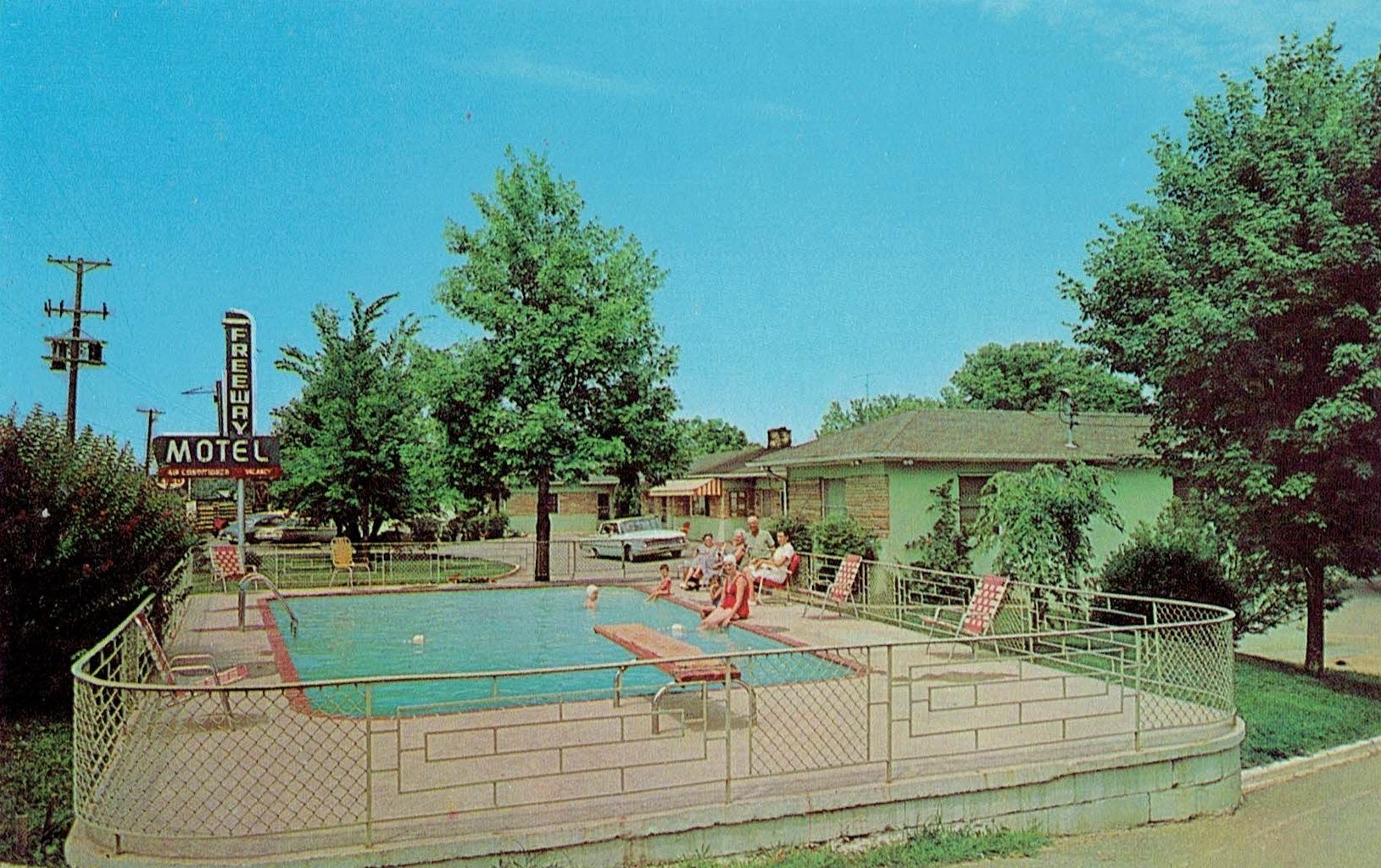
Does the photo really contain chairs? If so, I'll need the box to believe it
[802,554,863,619]
[753,555,800,606]
[135,613,248,740]
[921,575,1010,663]
[209,545,257,594]
[329,537,371,589]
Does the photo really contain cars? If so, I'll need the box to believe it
[577,515,689,562]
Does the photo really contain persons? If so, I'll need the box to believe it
[582,516,793,630]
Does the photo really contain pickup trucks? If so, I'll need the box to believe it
[217,511,338,544]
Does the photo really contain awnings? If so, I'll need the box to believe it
[650,478,722,496]
